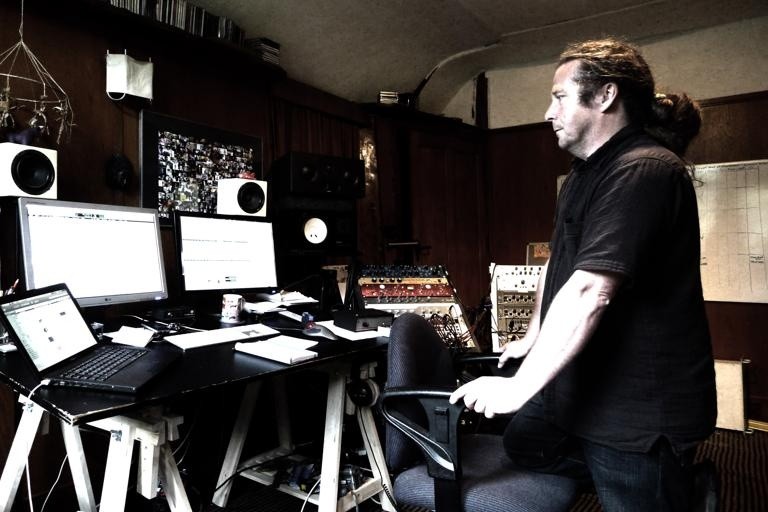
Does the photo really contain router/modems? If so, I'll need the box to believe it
[334,308,394,332]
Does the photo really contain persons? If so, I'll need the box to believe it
[448,34,717,512]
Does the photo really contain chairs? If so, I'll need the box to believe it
[377,313,582,512]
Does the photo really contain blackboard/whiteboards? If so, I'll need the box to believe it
[557,159,768,303]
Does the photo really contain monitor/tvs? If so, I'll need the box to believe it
[173,211,281,330]
[17,197,169,340]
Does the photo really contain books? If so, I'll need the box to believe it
[111,0,281,67]
[103,325,155,348]
[242,290,319,315]
[378,90,400,105]
[235,335,319,365]
[314,308,394,342]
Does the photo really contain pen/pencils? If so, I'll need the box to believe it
[0,279,19,297]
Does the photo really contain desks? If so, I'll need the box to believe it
[1,314,388,511]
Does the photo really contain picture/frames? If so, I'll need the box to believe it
[138,108,264,226]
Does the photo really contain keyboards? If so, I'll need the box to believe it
[163,322,280,350]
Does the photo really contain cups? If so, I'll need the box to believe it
[221,294,243,324]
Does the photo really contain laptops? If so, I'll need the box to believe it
[0,283,183,395]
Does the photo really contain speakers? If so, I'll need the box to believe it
[216,178,268,217]
[274,151,365,198]
[0,141,58,200]
[287,209,344,256]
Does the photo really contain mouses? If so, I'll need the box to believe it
[302,321,339,341]
